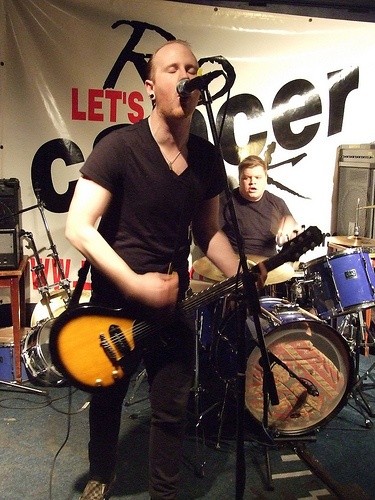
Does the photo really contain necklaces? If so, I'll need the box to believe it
[160,147,187,171]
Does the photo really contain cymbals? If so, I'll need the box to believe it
[326,236,375,246]
[193,253,294,286]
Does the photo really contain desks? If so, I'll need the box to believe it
[0,256,30,386]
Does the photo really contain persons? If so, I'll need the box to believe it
[218,154,317,316]
[64,37,267,500]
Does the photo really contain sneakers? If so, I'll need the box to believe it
[78,472,116,500]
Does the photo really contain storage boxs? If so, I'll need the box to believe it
[0,346,29,382]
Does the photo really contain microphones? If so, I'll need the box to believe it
[177,70,224,99]
[307,385,319,396]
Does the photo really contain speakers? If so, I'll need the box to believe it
[329,144,375,241]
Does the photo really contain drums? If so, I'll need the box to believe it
[301,245,375,319]
[22,317,68,387]
[211,295,356,440]
[0,325,30,384]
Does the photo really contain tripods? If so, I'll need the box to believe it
[202,338,318,490]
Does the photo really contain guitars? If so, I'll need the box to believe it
[48,224,330,390]
